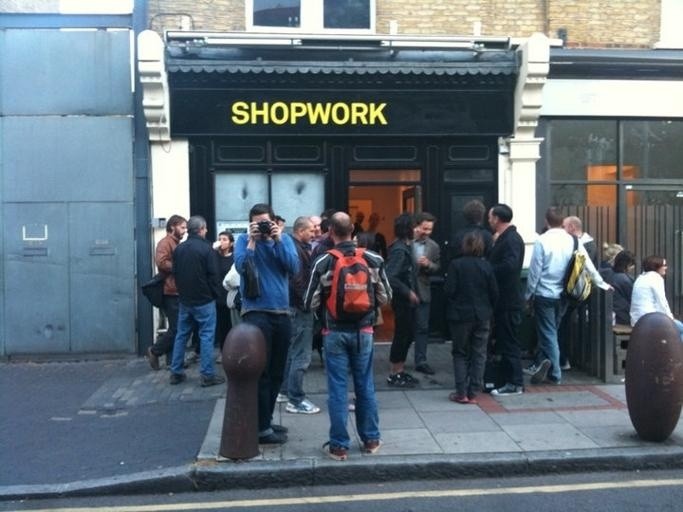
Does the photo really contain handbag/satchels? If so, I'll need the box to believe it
[564,252,591,302]
[141,273,164,308]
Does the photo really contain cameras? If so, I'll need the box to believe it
[256,220,271,235]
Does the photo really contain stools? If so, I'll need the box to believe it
[613,324,634,374]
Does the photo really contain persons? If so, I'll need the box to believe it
[302,212,393,461]
[272,215,329,415]
[148,215,233,388]
[597,241,624,284]
[610,250,636,325]
[233,203,301,444]
[629,255,683,332]
[353,202,613,404]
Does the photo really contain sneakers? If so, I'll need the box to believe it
[258,389,383,460]
[386,360,571,401]
[147,346,226,386]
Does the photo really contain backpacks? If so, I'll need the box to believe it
[325,248,374,322]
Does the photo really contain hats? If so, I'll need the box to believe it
[603,242,623,264]
